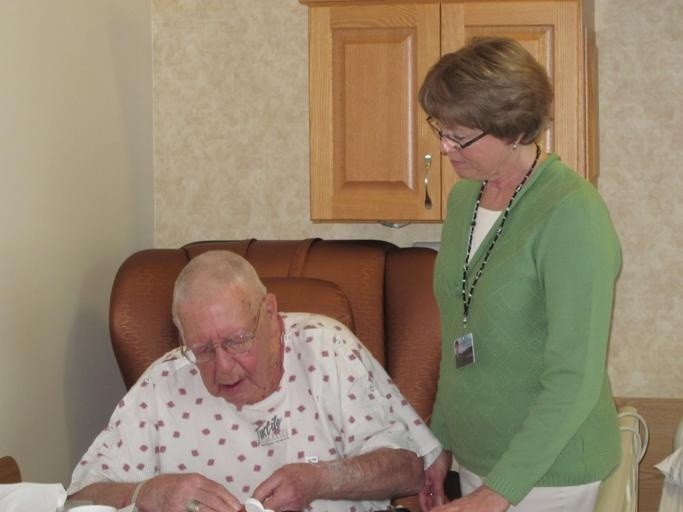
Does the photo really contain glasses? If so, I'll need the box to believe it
[179,311,260,366]
[425,116,488,151]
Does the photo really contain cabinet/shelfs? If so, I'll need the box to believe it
[298,0,602,227]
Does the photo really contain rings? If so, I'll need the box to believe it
[186,500,200,511]
[430,493,433,496]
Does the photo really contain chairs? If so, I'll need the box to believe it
[108,238,441,512]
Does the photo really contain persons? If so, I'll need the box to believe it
[454,341,466,368]
[417,36,623,512]
[65,249,442,511]
